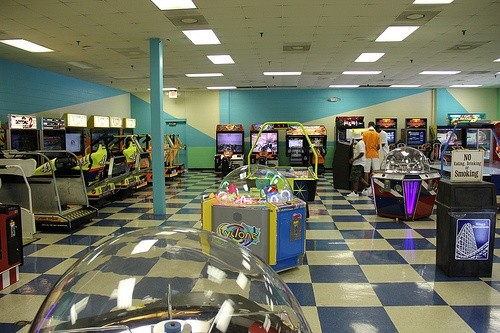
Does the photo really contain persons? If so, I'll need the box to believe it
[347,132,372,197]
[374,125,389,164]
[361,121,381,195]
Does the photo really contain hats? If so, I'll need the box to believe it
[354,132,363,139]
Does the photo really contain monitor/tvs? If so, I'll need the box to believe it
[287,137,304,149]
[407,130,425,145]
[385,131,395,144]
[250,131,278,153]
[10,130,106,155]
[217,132,244,154]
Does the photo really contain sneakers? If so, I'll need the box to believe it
[361,186,372,195]
[347,191,359,196]
